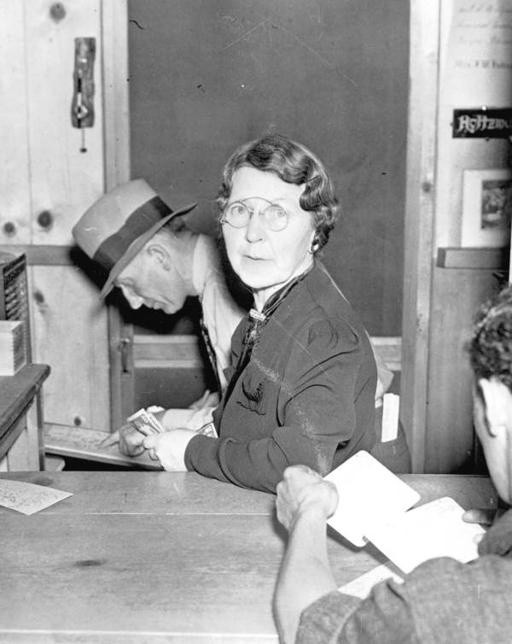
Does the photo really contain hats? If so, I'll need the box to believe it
[69,177,200,309]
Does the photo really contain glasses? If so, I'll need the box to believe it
[216,196,291,232]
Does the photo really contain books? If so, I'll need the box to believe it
[43,423,163,469]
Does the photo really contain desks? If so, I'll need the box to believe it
[0,469,498,643]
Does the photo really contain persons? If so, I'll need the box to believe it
[74,178,412,475]
[273,287,512,643]
[141,133,377,494]
